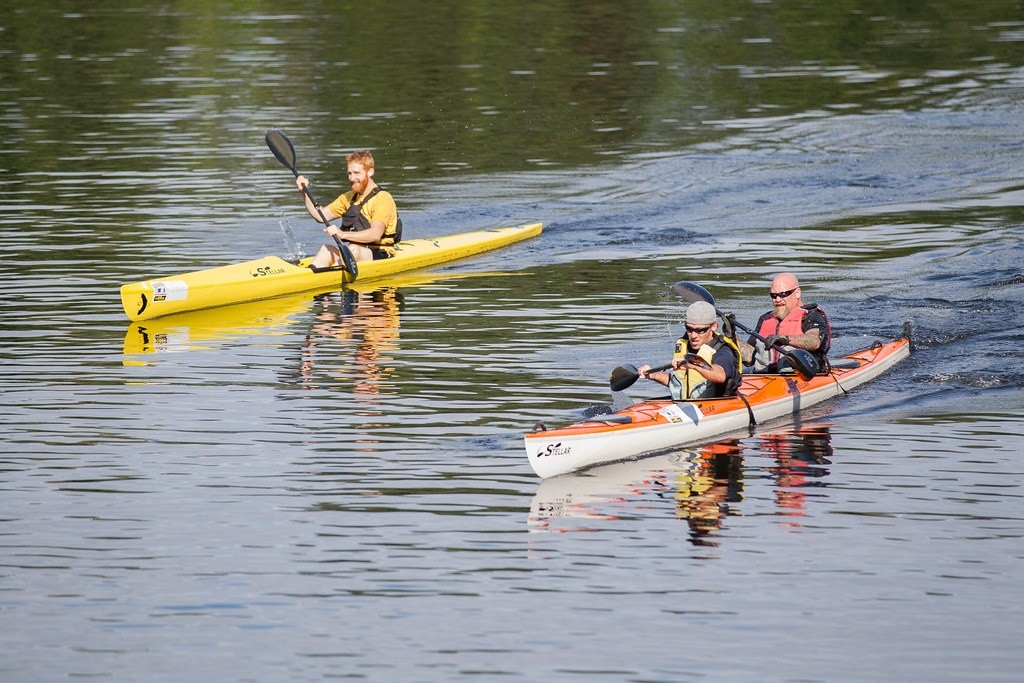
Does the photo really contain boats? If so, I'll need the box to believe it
[522,321,916,479]
[120,223,542,323]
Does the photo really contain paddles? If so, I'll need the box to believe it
[609,352,713,390]
[265,128,359,278]
[673,282,820,382]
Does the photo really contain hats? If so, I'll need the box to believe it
[685,299,717,326]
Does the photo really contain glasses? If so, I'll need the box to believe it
[684,322,712,335]
[769,288,796,299]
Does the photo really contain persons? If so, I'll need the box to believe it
[296,151,402,268]
[721,272,831,374]
[638,301,742,400]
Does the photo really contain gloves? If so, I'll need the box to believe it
[722,310,737,338]
[764,333,791,351]
